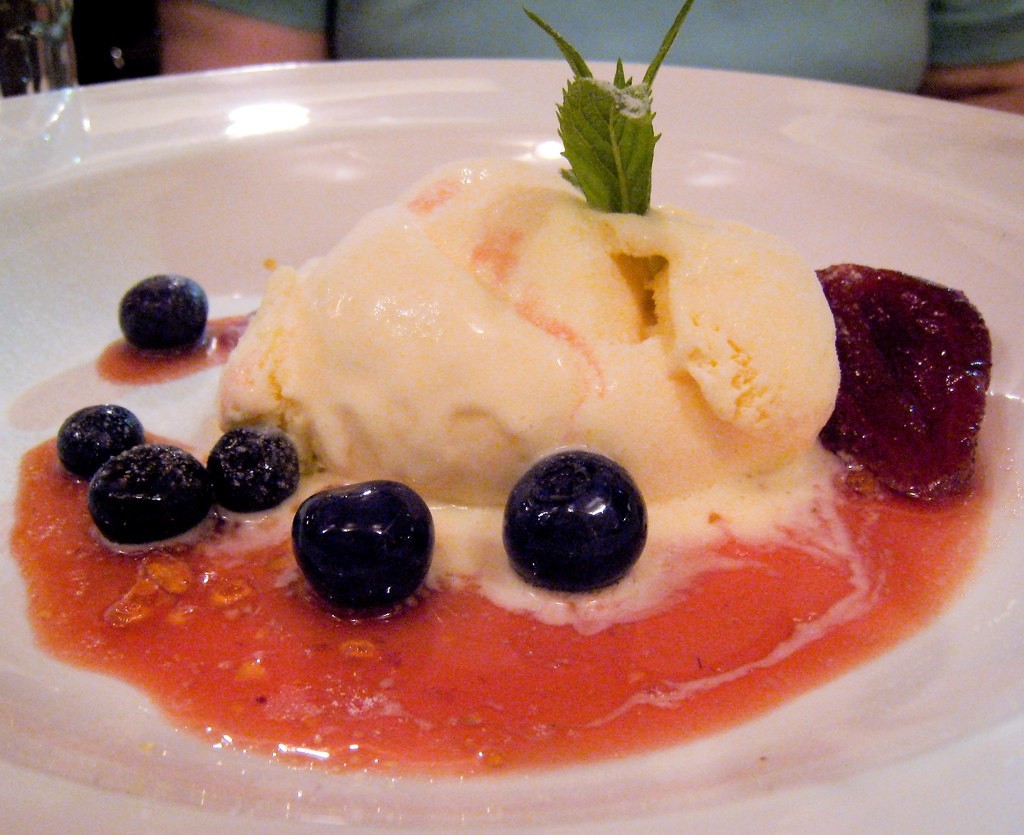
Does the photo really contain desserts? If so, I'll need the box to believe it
[220,162,842,509]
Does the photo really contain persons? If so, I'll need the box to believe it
[161,0,1024,113]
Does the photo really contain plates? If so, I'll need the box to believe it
[0,60,1024,835]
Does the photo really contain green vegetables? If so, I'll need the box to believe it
[521,0,696,214]
